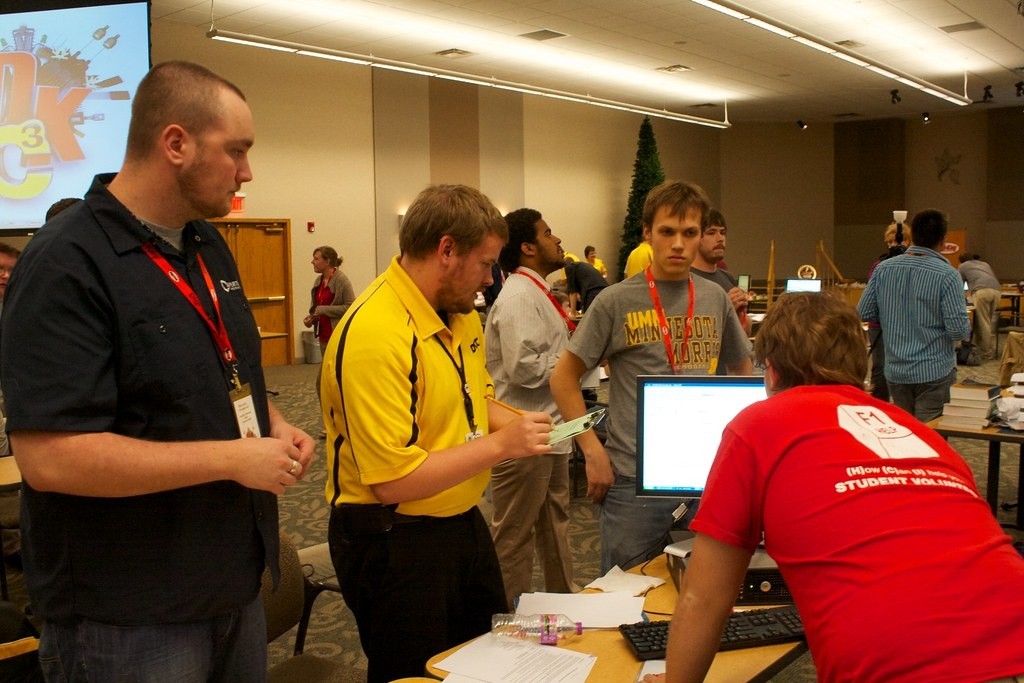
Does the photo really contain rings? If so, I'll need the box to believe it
[289,461,298,474]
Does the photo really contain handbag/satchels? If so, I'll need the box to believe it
[955,340,981,366]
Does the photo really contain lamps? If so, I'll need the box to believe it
[891,89,900,103]
[693,0,975,108]
[797,119,808,130]
[923,112,930,124]
[204,19,734,131]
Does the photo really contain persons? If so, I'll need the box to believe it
[317,184,554,682]
[0,62,317,683]
[866,223,913,404]
[640,292,1023,683]
[687,208,749,375]
[956,252,1000,360]
[304,245,356,438]
[549,178,756,576]
[0,197,83,309]
[481,208,583,615]
[477,235,654,322]
[855,208,971,424]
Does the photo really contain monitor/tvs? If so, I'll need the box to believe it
[636,374,769,559]
[785,278,822,293]
[736,274,751,293]
[963,281,969,291]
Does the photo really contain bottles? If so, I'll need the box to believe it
[491,613,583,643]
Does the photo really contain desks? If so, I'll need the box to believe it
[424,553,809,683]
[925,415,1023,542]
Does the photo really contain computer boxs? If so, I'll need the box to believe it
[665,546,796,607]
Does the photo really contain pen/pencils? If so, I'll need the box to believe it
[484,395,559,431]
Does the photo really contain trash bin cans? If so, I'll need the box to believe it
[303,331,323,364]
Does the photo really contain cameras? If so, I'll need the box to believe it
[888,210,908,258]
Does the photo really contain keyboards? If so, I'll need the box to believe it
[619,606,805,662]
